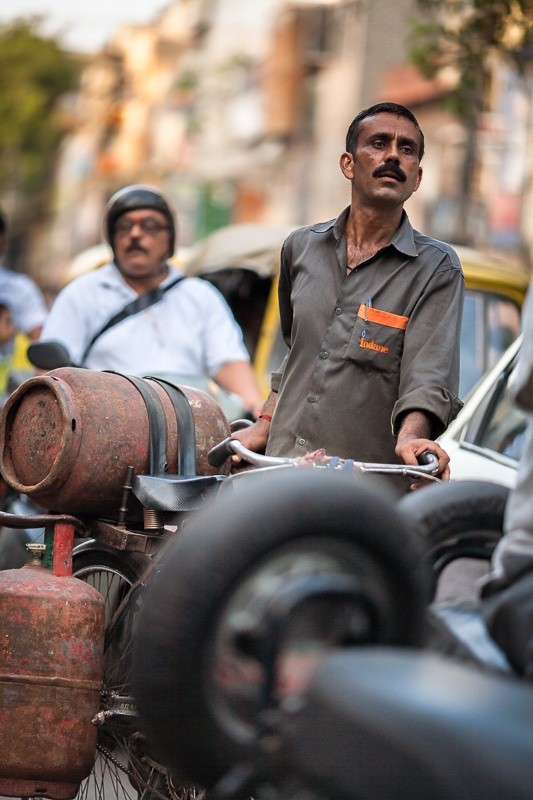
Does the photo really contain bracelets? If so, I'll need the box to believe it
[259,414,272,422]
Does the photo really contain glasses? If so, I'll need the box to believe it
[114,216,171,235]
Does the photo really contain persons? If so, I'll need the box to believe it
[231,102,465,499]
[0,185,265,570]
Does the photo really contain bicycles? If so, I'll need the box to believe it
[69,418,448,800]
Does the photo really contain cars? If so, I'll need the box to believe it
[0,226,533,603]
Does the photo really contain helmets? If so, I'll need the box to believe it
[103,185,175,256]
